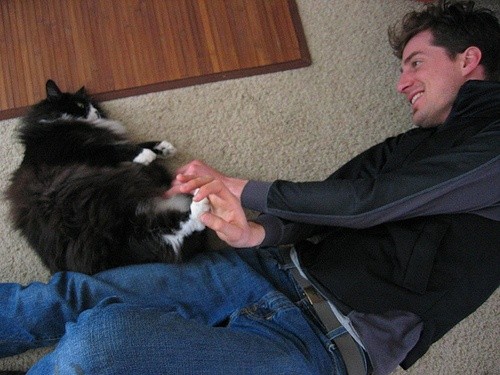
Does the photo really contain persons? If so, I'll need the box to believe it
[0,0,500,375]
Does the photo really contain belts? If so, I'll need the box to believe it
[277,245,367,375]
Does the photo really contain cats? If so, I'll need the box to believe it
[1,78,210,281]
